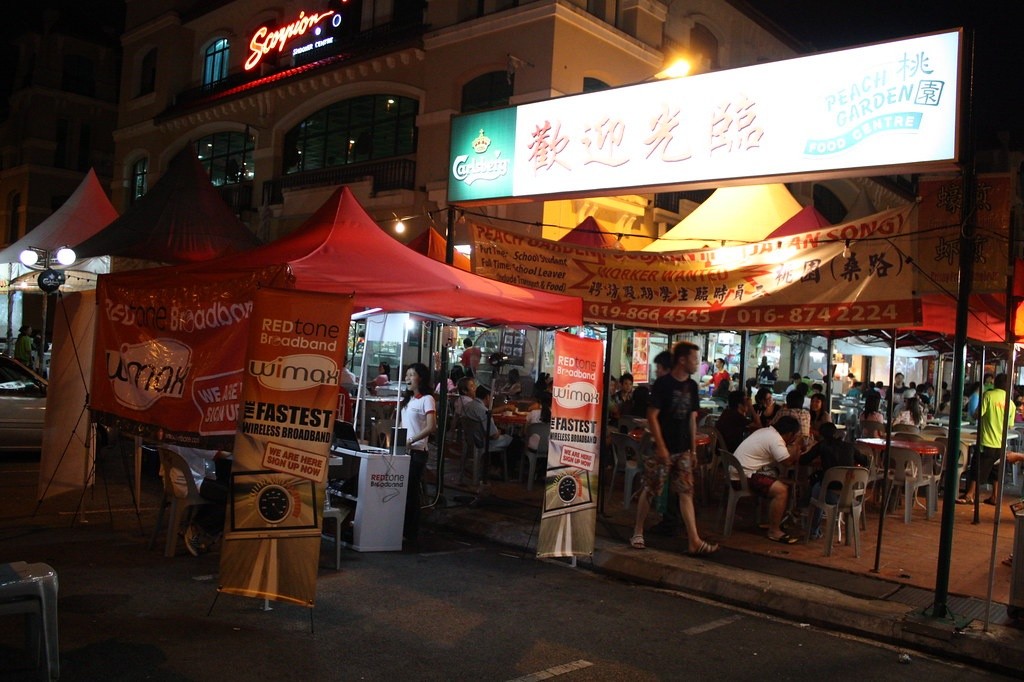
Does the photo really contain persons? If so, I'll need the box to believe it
[339,356,356,397]
[460,338,481,377]
[711,366,1024,543]
[630,340,720,555]
[701,356,709,377]
[14,324,33,366]
[608,373,634,420]
[159,444,231,556]
[702,358,730,390]
[366,362,391,396]
[436,365,552,451]
[30,329,49,379]
[399,363,436,541]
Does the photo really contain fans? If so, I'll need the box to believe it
[469,325,535,490]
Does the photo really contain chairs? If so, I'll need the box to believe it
[345,379,1023,559]
[147,447,214,558]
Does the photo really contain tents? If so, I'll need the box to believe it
[81,186,583,607]
[0,138,266,338]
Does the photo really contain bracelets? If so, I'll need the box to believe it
[409,437,414,443]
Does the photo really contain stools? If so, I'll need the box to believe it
[323,507,345,569]
[1,562,60,682]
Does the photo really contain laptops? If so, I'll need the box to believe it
[333,419,385,454]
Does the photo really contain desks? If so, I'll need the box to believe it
[961,424,1018,440]
[492,411,529,436]
[853,437,939,512]
[928,418,972,427]
[628,427,712,450]
[349,395,405,446]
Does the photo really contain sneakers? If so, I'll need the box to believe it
[182,520,200,556]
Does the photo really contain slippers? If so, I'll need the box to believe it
[685,541,719,556]
[983,498,997,506]
[628,534,646,549]
[955,495,975,505]
[768,532,799,544]
